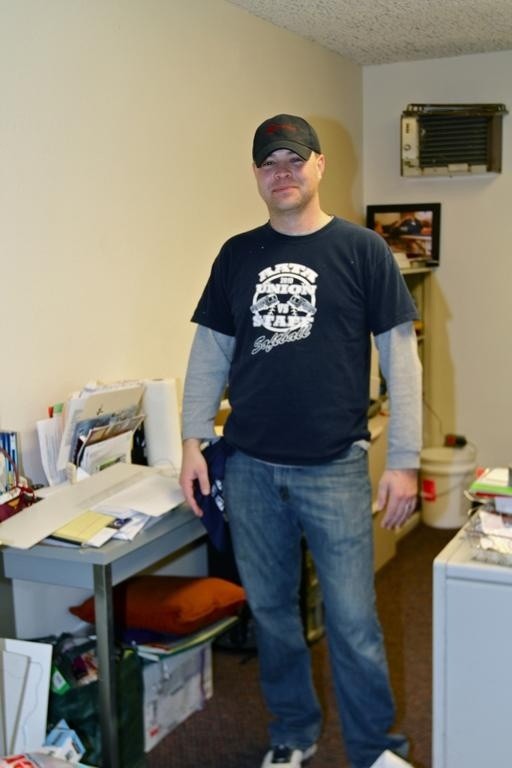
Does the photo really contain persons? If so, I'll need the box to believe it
[179,114,425,768]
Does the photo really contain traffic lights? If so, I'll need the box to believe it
[70,574,245,636]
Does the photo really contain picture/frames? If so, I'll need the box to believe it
[1,496,210,768]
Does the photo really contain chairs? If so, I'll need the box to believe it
[261,744,318,768]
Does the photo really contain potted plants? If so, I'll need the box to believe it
[364,202,443,266]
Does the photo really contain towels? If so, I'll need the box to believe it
[26,637,145,768]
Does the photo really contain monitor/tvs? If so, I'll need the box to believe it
[137,616,237,662]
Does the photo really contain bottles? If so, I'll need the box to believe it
[252,115,322,169]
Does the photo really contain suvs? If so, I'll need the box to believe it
[421,446,476,529]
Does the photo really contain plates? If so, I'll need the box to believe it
[369,268,434,402]
[430,504,512,768]
[365,406,423,575]
